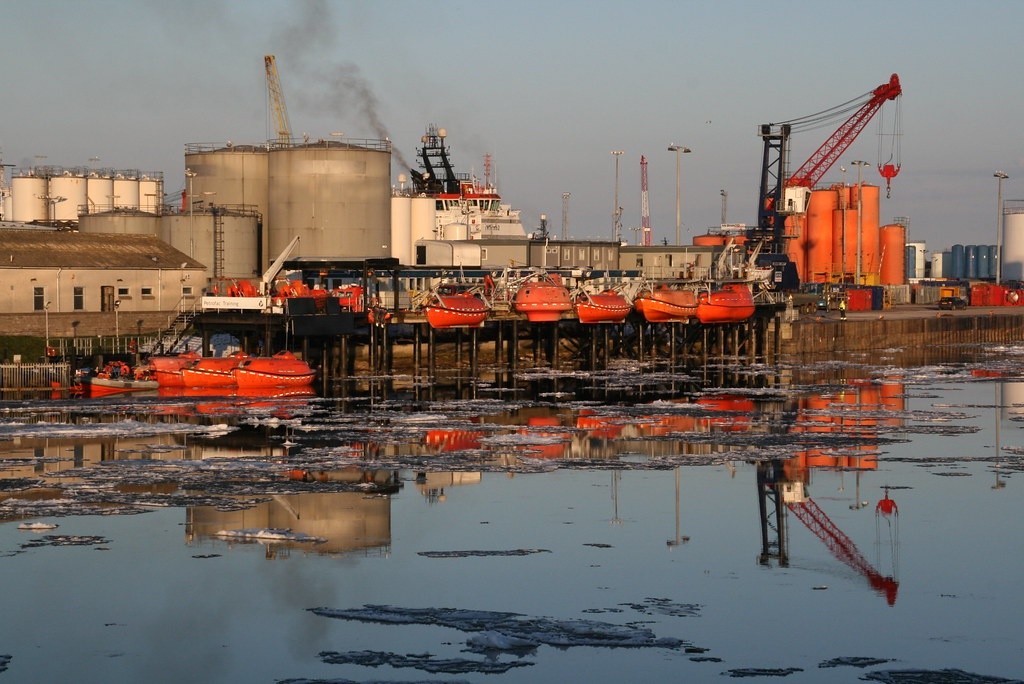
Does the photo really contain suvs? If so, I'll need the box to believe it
[939,297,967,311]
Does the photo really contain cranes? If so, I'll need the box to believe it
[778,467,900,607]
[693,71,904,254]
[265,53,296,150]
[639,155,653,247]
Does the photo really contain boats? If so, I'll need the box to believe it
[577,289,632,325]
[635,287,699,324]
[425,294,491,328]
[514,280,574,322]
[695,281,756,325]
[88,349,318,398]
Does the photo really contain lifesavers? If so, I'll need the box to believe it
[47,347,56,356]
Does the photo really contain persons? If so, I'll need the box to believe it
[128,337,138,354]
[839,298,847,320]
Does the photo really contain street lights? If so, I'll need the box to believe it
[183,166,198,261]
[45,301,50,348]
[667,143,692,246]
[994,170,1010,287]
[609,150,624,243]
[113,300,121,354]
